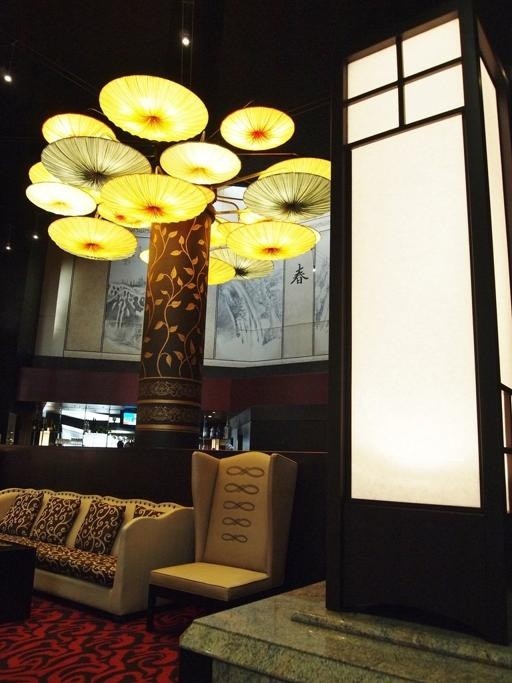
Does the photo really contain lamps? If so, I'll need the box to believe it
[339,17,511,506]
[26,74,331,285]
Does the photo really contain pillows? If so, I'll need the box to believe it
[0,493,166,551]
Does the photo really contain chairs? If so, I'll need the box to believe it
[148,451,301,636]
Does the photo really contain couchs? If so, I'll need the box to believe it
[0,487,197,616]
